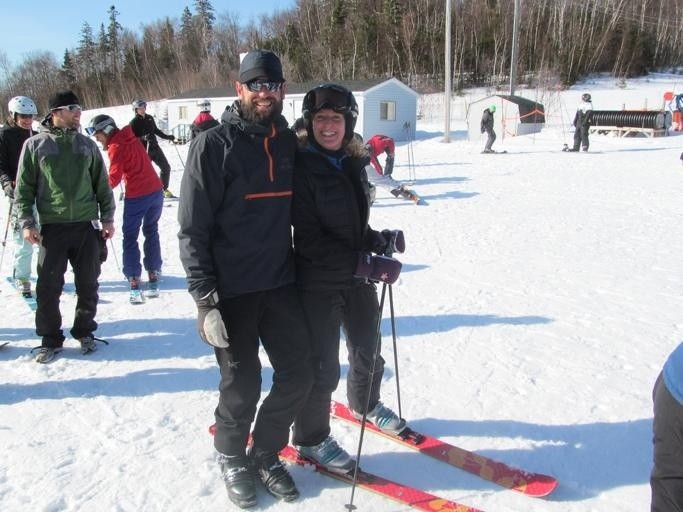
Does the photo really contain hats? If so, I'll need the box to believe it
[49,90,79,108]
[238,50,285,83]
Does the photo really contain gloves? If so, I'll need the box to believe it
[354,250,402,283]
[196,290,230,350]
[2,181,14,198]
[168,134,175,141]
[377,229,406,254]
[144,134,153,142]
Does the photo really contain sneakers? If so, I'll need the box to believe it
[484,148,495,152]
[78,335,98,354]
[568,147,589,152]
[352,403,406,435]
[129,278,142,291]
[216,448,257,508]
[163,189,172,197]
[148,268,159,286]
[294,436,356,473]
[249,449,297,502]
[36,342,64,363]
[15,276,32,294]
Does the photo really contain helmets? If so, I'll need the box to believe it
[132,99,147,112]
[88,115,117,136]
[582,92,591,102]
[490,105,496,113]
[197,99,211,113]
[302,83,359,136]
[8,95,39,121]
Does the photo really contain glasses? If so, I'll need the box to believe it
[50,105,83,113]
[304,87,352,112]
[247,80,283,92]
[16,113,33,120]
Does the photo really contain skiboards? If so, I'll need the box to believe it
[480,151,526,155]
[208,400,557,511]
[7,273,157,312]
[391,188,422,207]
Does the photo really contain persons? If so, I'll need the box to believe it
[14,90,115,363]
[365,136,395,176]
[568,93,592,152]
[666,92,683,132]
[177,45,315,506]
[481,105,495,152]
[0,95,46,291]
[85,114,165,288]
[131,101,175,196]
[190,97,217,139]
[651,340,683,512]
[291,86,410,469]
